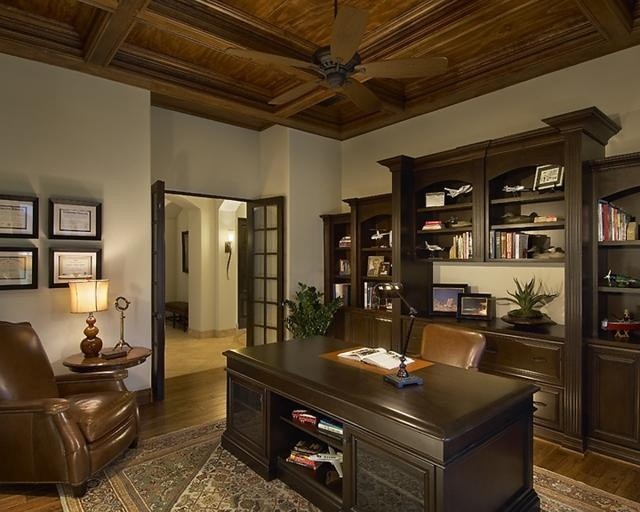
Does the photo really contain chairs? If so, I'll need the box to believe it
[0,319,140,498]
[420,323,486,371]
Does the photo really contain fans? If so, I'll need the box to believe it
[223,0,450,117]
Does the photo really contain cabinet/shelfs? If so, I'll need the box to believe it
[342,307,401,350]
[581,340,639,464]
[401,316,580,450]
[348,196,400,312]
[323,213,353,338]
[592,152,639,350]
[374,104,625,266]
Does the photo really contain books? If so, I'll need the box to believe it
[337,346,415,370]
[448,231,473,260]
[318,416,343,442]
[489,230,549,259]
[286,440,328,470]
[339,256,351,275]
[334,283,351,306]
[364,281,380,309]
[101,350,127,360]
[597,202,639,241]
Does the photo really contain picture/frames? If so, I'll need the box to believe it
[48,198,103,241]
[532,164,564,191]
[456,292,492,320]
[47,246,103,290]
[426,283,469,318]
[0,246,38,291]
[181,231,188,274]
[0,194,40,239]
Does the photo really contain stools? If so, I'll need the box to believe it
[176,300,188,332]
[166,301,184,328]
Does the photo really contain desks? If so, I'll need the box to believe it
[222,336,541,512]
[62,346,152,389]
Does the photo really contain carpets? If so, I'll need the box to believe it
[54,417,639,511]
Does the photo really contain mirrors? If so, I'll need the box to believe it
[111,296,133,352]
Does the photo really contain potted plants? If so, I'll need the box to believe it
[496,275,559,327]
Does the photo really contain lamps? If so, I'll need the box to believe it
[225,231,233,280]
[369,281,425,391]
[69,278,107,360]
